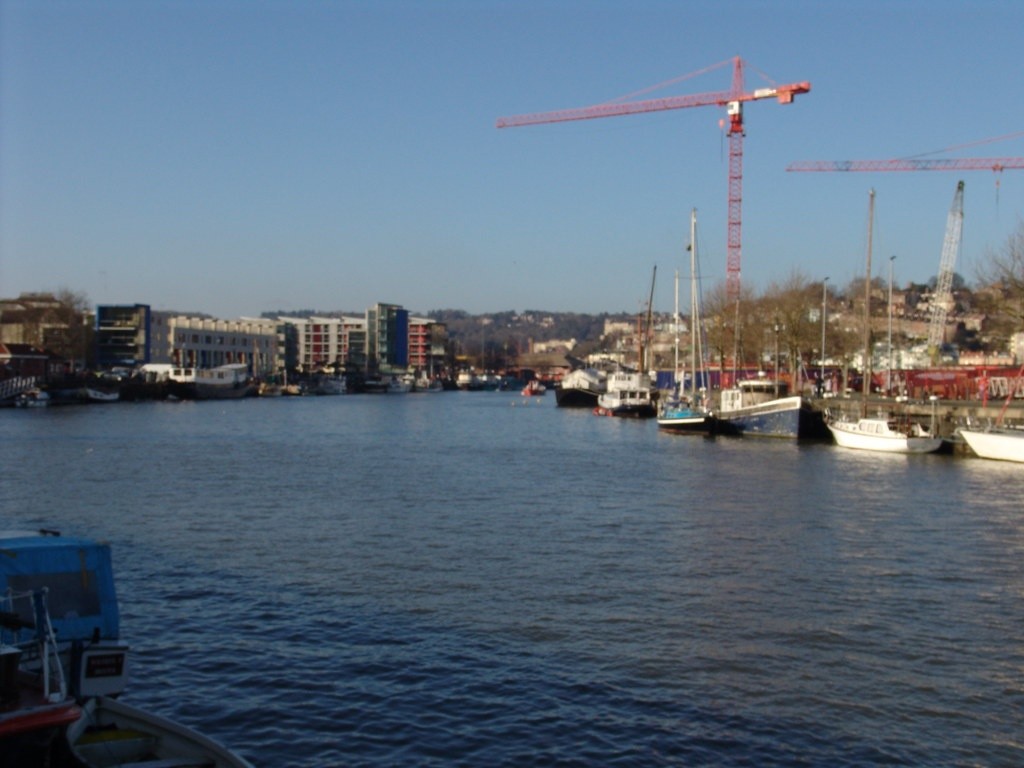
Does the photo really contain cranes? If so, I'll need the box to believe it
[788,132,1024,170]
[494,55,812,388]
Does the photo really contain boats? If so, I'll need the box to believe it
[596,371,655,416]
[0,524,255,768]
[1,356,509,414]
[821,394,943,454]
[958,428,1024,463]
[552,367,609,407]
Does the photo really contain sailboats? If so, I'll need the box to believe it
[657,206,715,428]
[714,296,812,439]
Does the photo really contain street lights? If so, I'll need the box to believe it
[888,255,897,397]
[821,275,832,392]
